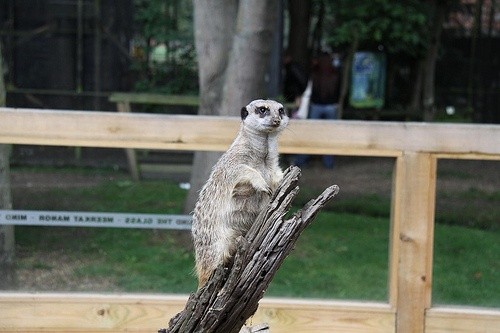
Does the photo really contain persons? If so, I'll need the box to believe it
[289,51,341,171]
